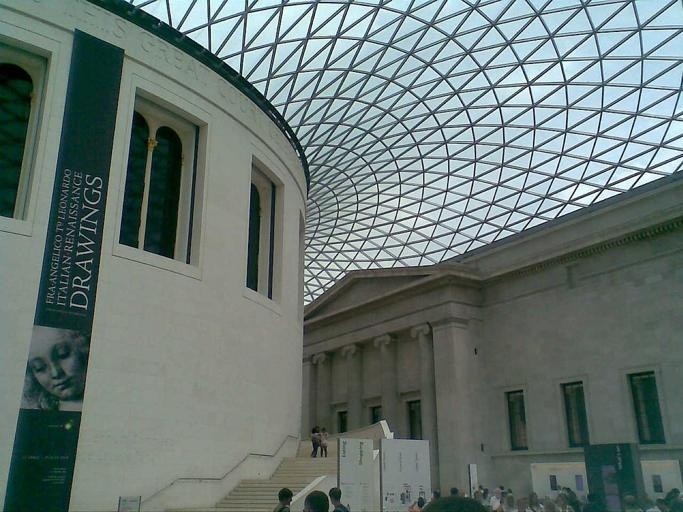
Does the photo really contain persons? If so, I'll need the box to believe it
[328,487,348,512]
[19,325,89,411]
[302,490,329,512]
[309,424,329,458]
[407,485,682,512]
[272,488,293,512]
